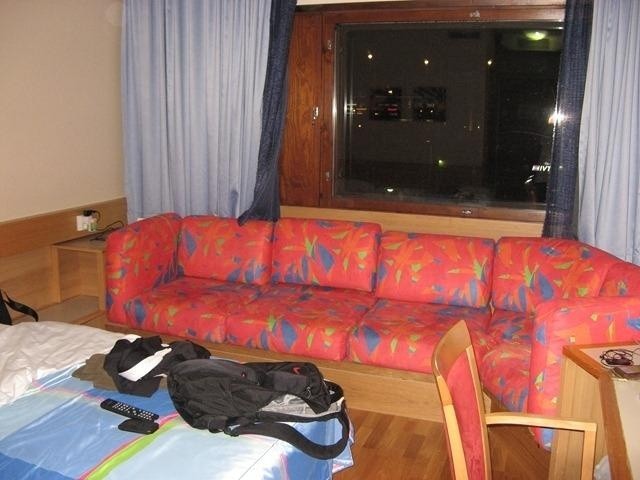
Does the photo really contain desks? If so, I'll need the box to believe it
[50,233,109,312]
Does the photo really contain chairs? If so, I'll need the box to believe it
[430,318,599,480]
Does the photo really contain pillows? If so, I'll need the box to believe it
[494,235,587,310]
[376,229,494,307]
[273,214,383,294]
[178,213,274,286]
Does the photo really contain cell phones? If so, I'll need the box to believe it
[118,418,159,434]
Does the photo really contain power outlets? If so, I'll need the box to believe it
[75,214,93,231]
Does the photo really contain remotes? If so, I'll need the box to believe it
[100,398,159,422]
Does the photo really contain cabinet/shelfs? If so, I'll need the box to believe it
[548,340,640,480]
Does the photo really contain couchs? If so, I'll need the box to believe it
[104,212,640,454]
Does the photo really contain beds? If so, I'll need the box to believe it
[0,317,348,480]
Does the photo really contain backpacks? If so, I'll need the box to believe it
[167,359,349,460]
